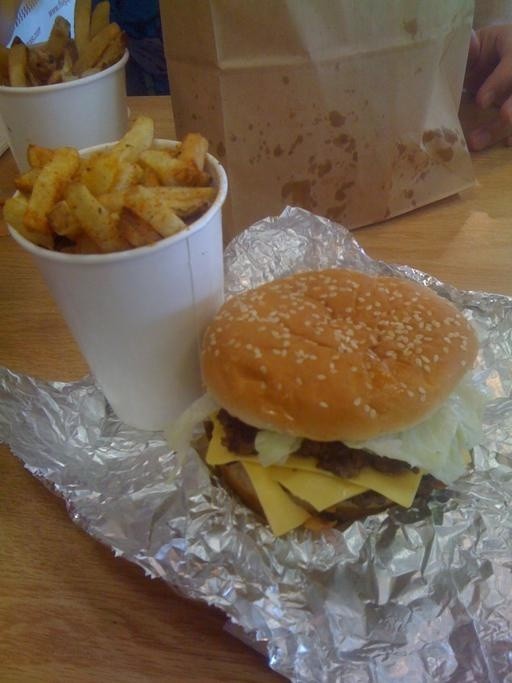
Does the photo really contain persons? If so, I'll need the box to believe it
[458,23,512,151]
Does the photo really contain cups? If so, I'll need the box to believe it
[5,136,231,434]
[0,46,132,184]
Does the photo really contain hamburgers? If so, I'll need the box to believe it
[201,269,480,538]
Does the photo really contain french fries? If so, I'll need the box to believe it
[4,116,217,254]
[0,1,129,86]
